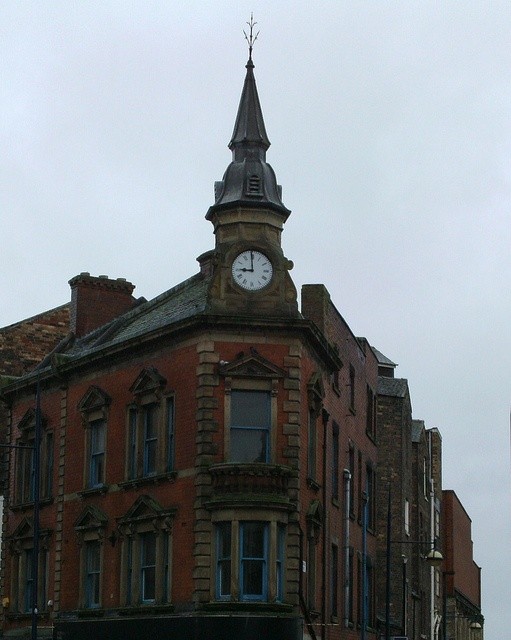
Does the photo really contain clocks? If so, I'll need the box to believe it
[225,241,282,296]
